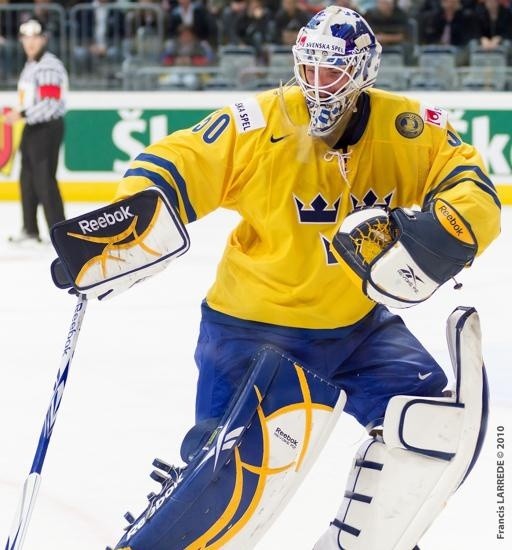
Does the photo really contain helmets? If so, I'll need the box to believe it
[296,6,382,90]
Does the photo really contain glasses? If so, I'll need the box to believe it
[18,19,43,36]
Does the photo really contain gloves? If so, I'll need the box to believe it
[362,199,478,309]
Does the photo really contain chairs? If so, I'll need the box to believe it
[205,18,512,92]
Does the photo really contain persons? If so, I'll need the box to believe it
[106,3,506,550]
[6,14,76,253]
